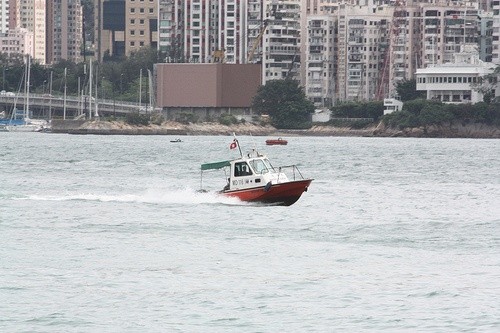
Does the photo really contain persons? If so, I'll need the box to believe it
[223,178,230,190]
[241,165,248,175]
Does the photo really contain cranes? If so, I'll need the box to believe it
[357,1,407,100]
[214,18,270,62]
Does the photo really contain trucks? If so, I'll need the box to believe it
[384,98,404,114]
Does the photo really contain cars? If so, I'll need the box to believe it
[42,93,54,97]
[77,95,96,101]
[3,91,14,97]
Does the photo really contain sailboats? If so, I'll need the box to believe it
[1,53,156,134]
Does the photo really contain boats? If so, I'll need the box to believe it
[196,132,313,207]
[266,138,288,146]
[169,138,183,143]
